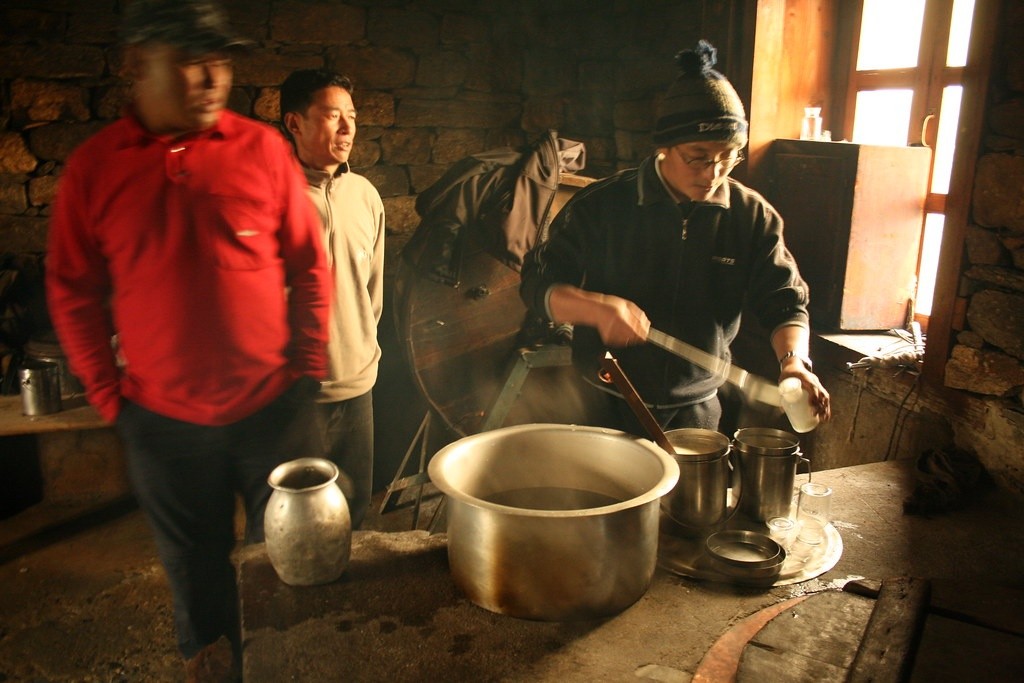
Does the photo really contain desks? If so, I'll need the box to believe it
[0,388,108,434]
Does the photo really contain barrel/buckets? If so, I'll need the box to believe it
[18,362,61,416]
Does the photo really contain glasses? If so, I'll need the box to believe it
[673,144,745,172]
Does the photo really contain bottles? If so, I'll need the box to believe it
[800,106,822,141]
[777,377,821,433]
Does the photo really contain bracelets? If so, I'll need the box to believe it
[780,350,812,372]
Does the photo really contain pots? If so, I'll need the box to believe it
[426,421,681,622]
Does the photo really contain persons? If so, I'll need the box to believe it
[279,68,386,530]
[519,39,832,438]
[46,19,330,683]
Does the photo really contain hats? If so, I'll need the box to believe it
[116,0,259,61]
[650,39,750,150]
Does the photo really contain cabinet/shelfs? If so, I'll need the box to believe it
[767,137,932,332]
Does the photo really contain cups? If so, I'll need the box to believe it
[650,428,735,540]
[795,481,834,556]
[729,428,812,523]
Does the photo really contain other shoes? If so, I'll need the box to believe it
[180,633,241,683]
